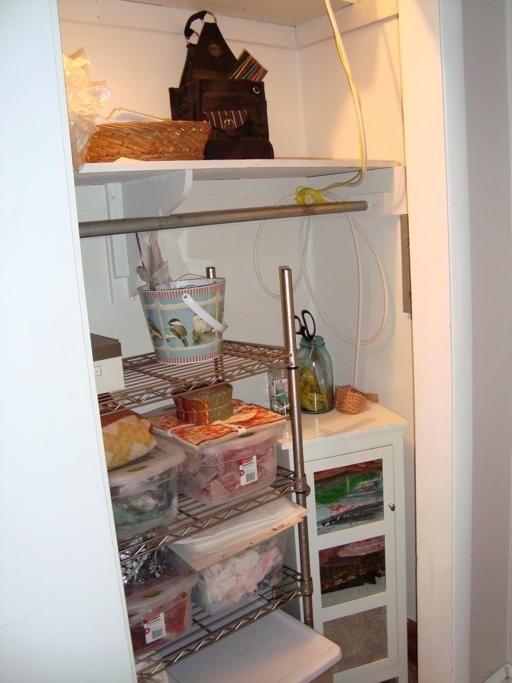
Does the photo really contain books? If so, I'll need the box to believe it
[124,550,199,657]
[142,398,286,506]
[108,434,187,541]
[149,487,307,613]
[90,331,125,395]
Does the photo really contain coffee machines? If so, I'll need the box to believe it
[294,309,329,411]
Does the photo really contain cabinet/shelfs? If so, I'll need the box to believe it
[298,336,337,414]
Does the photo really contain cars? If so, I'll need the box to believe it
[267,364,290,420]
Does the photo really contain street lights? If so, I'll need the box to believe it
[169,9,274,159]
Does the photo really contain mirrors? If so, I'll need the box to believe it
[166,317,190,348]
[190,313,205,343]
[145,318,169,344]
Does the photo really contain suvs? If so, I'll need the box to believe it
[137,277,230,365]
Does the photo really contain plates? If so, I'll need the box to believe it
[98,341,314,683]
[289,428,399,683]
